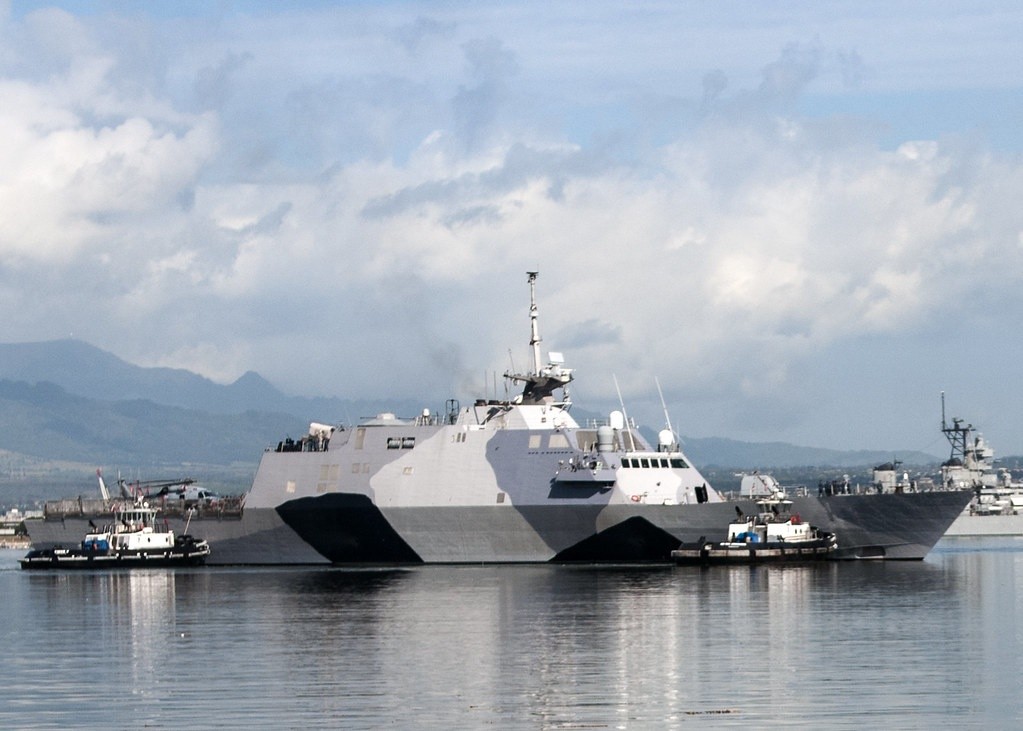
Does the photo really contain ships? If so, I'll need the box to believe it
[19,270,977,569]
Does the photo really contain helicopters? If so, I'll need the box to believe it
[107,468,221,515]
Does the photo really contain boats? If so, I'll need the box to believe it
[16,490,211,571]
[671,484,837,565]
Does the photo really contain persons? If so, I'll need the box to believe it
[218,492,244,515]
[877,480,884,496]
[306,429,335,451]
[908,477,916,494]
[819,479,850,495]
